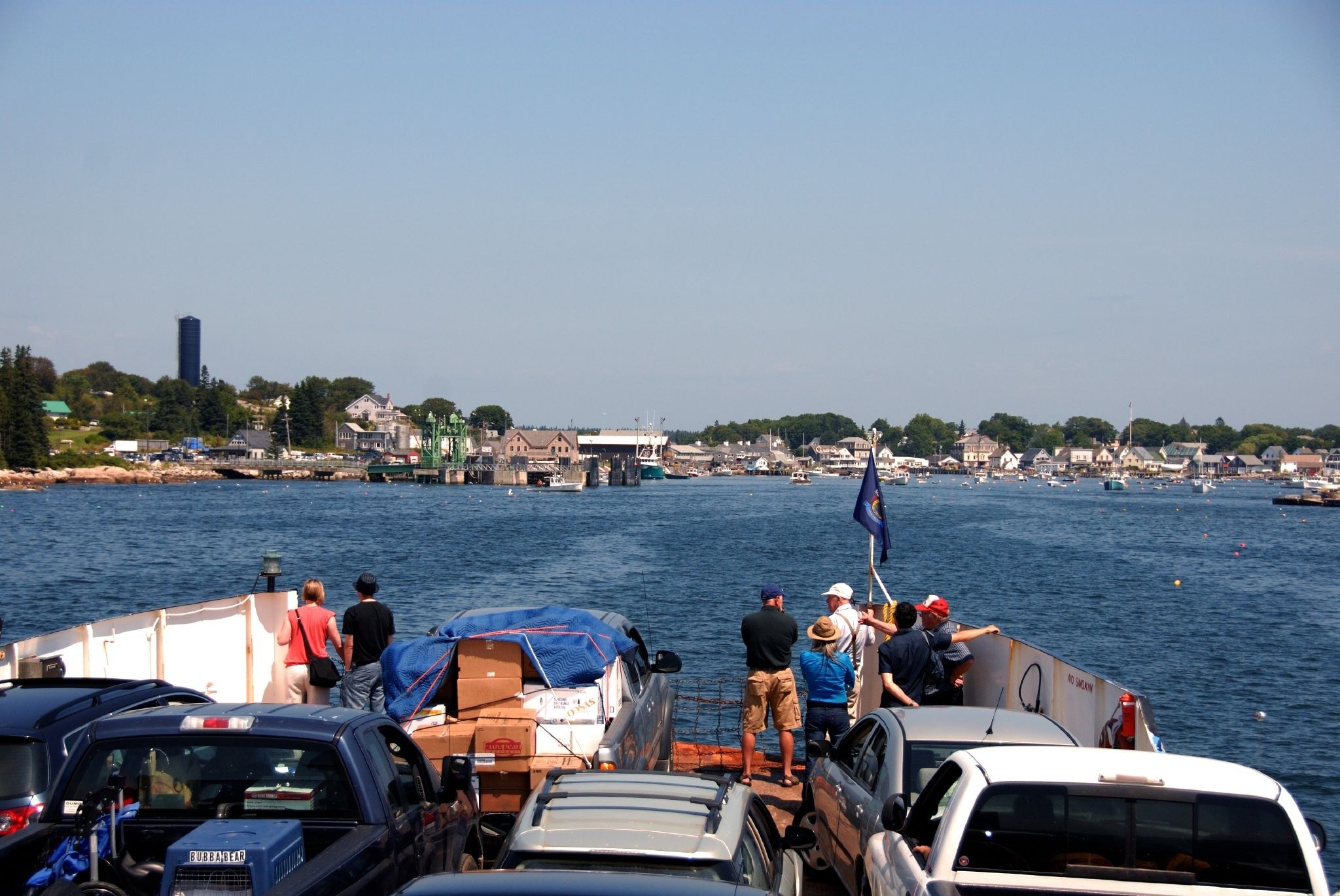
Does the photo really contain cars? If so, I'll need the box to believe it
[84,444,203,462]
[315,453,403,464]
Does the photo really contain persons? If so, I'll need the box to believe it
[741,584,802,787]
[802,583,1000,787]
[276,579,344,705]
[341,573,397,715]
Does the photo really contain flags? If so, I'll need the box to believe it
[853,452,892,565]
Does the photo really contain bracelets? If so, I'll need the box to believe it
[951,674,957,678]
[909,699,915,706]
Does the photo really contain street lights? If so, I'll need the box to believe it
[590,440,593,457]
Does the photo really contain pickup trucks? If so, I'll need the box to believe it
[396,601,685,822]
[861,741,1329,896]
[0,701,483,896]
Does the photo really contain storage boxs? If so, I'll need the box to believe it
[244,776,328,810]
[391,639,622,813]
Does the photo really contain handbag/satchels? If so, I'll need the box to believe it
[309,658,341,689]
[921,631,944,683]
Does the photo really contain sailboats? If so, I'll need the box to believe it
[1190,437,1216,493]
[1104,448,1126,490]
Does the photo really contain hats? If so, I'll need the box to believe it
[914,595,950,618]
[759,584,787,601]
[807,616,841,641]
[352,571,380,594]
[821,582,853,600]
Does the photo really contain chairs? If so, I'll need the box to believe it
[910,749,939,806]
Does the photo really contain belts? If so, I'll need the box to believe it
[750,666,787,671]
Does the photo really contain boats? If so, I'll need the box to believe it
[962,481,968,486]
[915,467,933,483]
[1102,471,1138,480]
[841,468,910,485]
[790,469,811,484]
[809,467,823,473]
[1280,470,1340,490]
[1218,478,1225,483]
[987,467,1004,480]
[1143,472,1184,490]
[710,465,732,476]
[974,466,988,483]
[1018,473,1078,487]
[636,409,699,480]
[525,472,583,492]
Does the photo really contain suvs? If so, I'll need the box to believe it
[502,767,812,896]
[0,678,214,844]
[806,704,1082,896]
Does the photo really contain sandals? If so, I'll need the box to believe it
[784,774,799,787]
[738,773,751,785]
